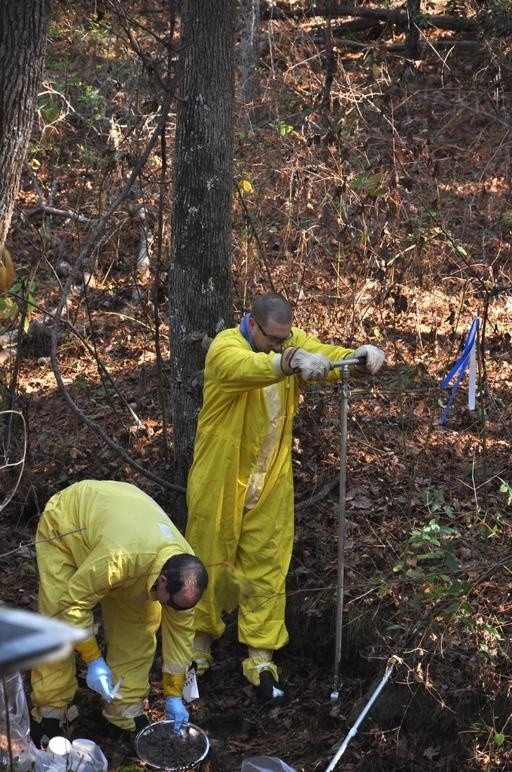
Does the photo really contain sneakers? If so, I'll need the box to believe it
[255,670,277,697]
[33,717,60,748]
[101,715,150,743]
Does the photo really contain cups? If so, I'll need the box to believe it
[72,738,98,752]
[44,734,72,755]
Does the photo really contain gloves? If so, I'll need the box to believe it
[343,344,385,377]
[281,345,334,381]
[86,657,112,703]
[165,696,189,733]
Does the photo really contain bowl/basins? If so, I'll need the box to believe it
[132,721,211,769]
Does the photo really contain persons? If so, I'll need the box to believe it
[187,292,387,709]
[27,479,208,749]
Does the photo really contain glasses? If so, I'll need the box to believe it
[253,318,293,341]
